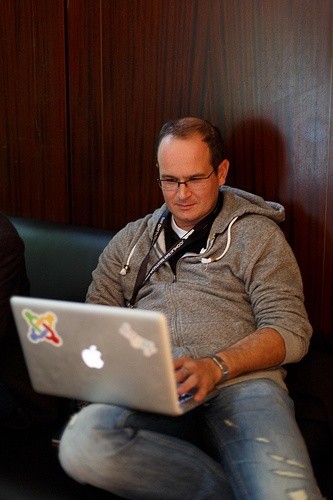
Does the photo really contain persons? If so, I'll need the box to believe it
[58,113,324,500]
[0,214,59,475]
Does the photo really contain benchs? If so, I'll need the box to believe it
[0,219,116,500]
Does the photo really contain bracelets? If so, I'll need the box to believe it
[204,354,228,383]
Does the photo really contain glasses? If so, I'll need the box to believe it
[157,170,218,191]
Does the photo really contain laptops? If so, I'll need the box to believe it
[10,296,220,416]
